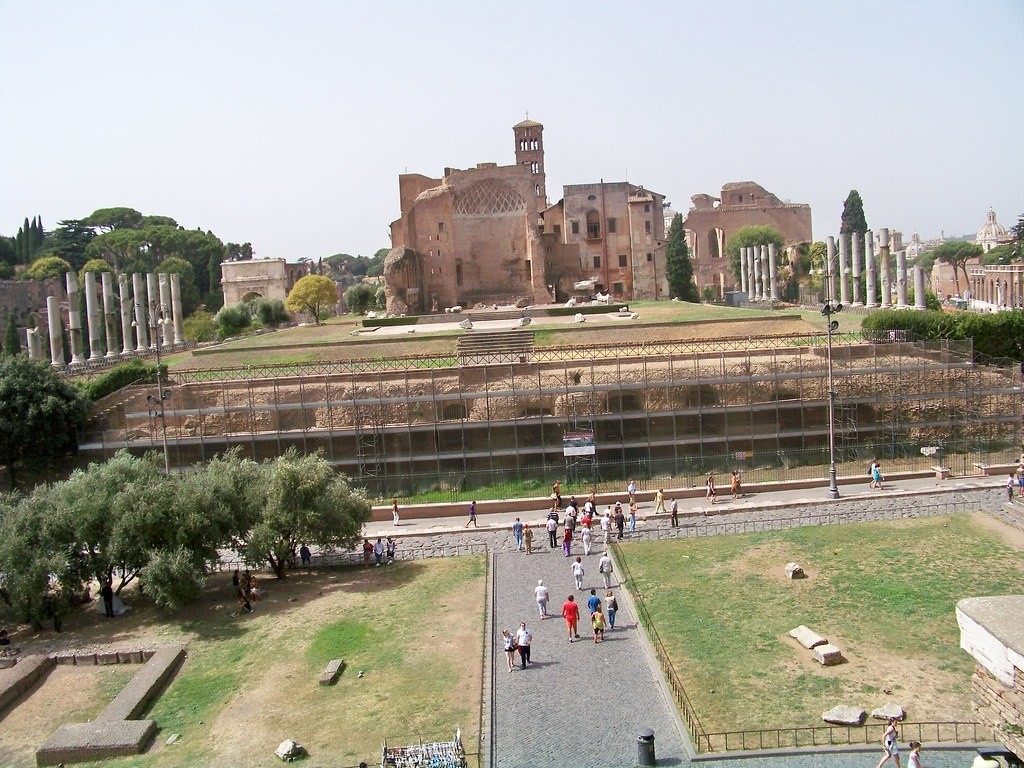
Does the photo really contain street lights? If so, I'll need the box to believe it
[130,303,173,475]
[808,250,852,500]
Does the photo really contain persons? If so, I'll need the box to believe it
[1007,454,1024,505]
[562,492,600,536]
[98,579,115,617]
[466,501,479,528]
[600,506,613,544]
[502,630,516,673]
[522,524,534,555]
[300,543,312,566]
[869,458,886,490]
[571,557,584,591]
[628,503,638,531]
[628,480,636,507]
[562,526,572,557]
[587,589,618,643]
[655,488,679,527]
[705,470,746,504]
[874,718,925,768]
[581,524,592,556]
[562,594,580,642]
[546,516,558,548]
[599,552,613,589]
[546,479,563,525]
[392,500,400,526]
[512,518,523,550]
[230,568,262,618]
[363,537,396,568]
[613,500,628,539]
[516,622,533,669]
[0,629,11,646]
[534,580,550,620]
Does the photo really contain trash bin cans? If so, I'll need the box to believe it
[636,727,655,765]
[981,308,983,313]
